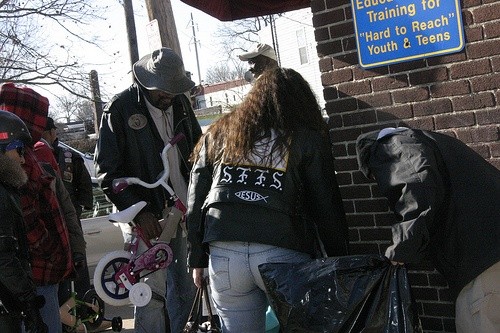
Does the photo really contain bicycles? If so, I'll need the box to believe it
[93,133,189,308]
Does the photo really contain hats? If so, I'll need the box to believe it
[237,42,279,63]
[0,83,49,145]
[132,48,195,95]
[47,116,58,129]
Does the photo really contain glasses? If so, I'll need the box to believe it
[16,147,25,157]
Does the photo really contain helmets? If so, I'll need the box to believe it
[0,109,32,147]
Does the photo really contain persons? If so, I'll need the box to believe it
[240,43,279,80]
[0,109,49,333]
[95,48,203,333]
[45,117,92,333]
[356,127,500,333]
[0,82,72,333]
[185,67,347,333]
[111,202,138,245]
